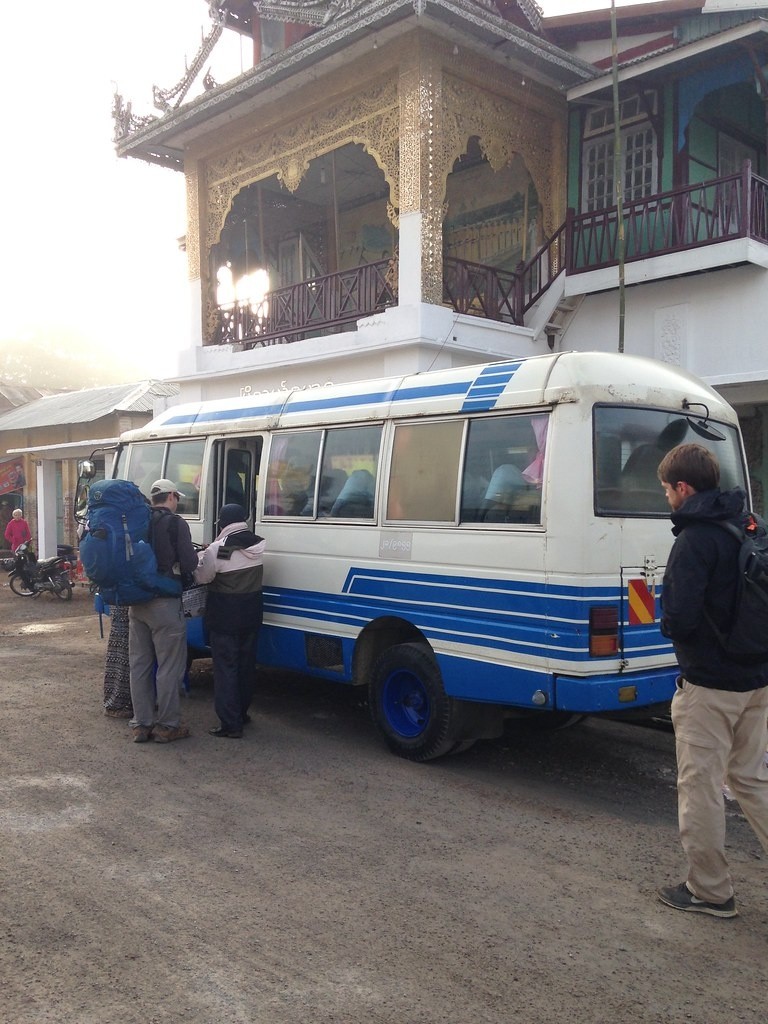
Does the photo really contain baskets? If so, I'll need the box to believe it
[0,552,15,571]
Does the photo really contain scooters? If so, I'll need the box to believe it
[0,537,77,601]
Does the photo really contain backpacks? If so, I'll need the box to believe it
[79,479,184,606]
[698,510,768,665]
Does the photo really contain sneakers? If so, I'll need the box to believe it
[656,881,738,917]
[133,726,151,743]
[151,724,188,742]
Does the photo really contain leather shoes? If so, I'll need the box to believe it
[208,725,242,737]
[240,713,251,724]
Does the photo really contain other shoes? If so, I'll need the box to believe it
[104,709,133,718]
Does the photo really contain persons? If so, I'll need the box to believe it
[657,443,768,916]
[4,509,31,556]
[101,478,266,743]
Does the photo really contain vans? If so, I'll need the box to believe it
[72,349,753,766]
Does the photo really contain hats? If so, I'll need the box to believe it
[214,504,247,525]
[149,479,185,497]
